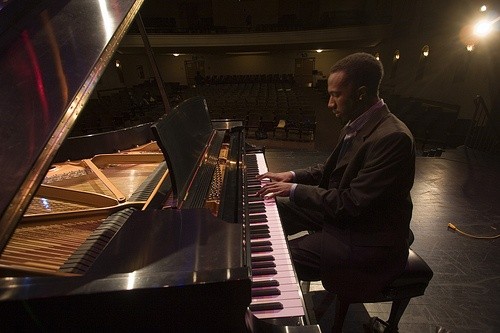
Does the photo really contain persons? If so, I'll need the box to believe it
[254,52,416,301]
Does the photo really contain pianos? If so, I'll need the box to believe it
[0,1,321,333]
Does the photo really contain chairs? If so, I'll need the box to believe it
[129,8,375,35]
[66,74,475,154]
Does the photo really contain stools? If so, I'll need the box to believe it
[321,248,434,333]
